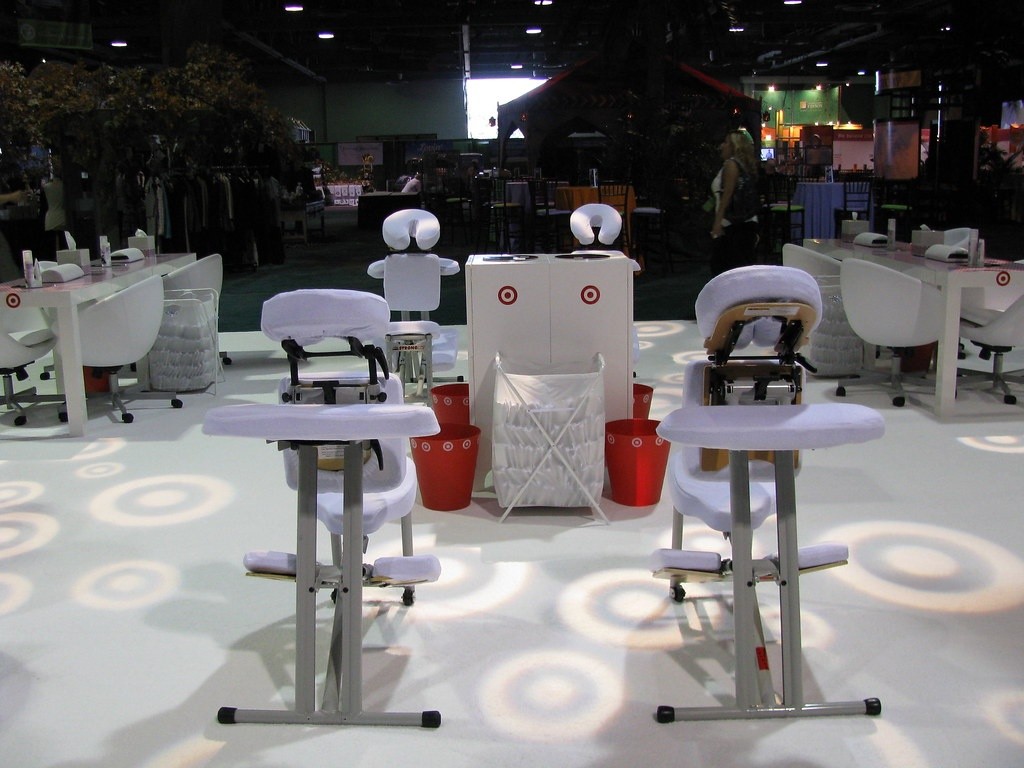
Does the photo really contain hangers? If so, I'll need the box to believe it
[208,164,241,178]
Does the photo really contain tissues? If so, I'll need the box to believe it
[841,211,870,244]
[127,228,156,257]
[911,224,945,258]
[55,230,92,276]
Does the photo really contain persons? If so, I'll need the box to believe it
[710,130,760,275]
[401,174,421,192]
[468,159,480,172]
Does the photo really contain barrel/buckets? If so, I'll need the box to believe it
[430,383,470,425]
[408,423,482,512]
[633,383,653,419]
[604,419,671,507]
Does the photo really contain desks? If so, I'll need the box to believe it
[0,253,196,435]
[805,238,1024,407]
[792,182,874,245]
[555,185,635,256]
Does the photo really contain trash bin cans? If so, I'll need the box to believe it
[411,422,482,511]
[604,418,670,508]
[430,383,469,424]
[633,383,654,418]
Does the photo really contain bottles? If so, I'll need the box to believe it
[100,235,108,267]
[968,229,980,267]
[887,219,896,250]
[23,251,34,287]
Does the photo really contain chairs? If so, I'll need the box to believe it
[782,244,880,361]
[50,274,164,423]
[835,256,958,406]
[957,259,1024,403]
[160,254,233,365]
[0,329,56,424]
[437,171,949,256]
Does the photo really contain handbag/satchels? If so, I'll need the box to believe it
[715,158,760,224]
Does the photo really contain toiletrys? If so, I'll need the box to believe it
[22,249,44,287]
[966,228,985,268]
[887,219,896,248]
[98,236,112,266]
